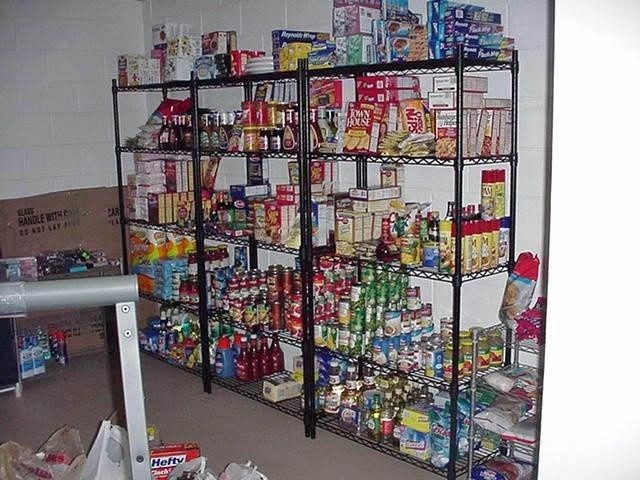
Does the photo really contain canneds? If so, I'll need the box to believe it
[422,242,440,267]
[242,98,287,152]
[378,418,393,442]
[400,234,421,266]
[222,256,504,384]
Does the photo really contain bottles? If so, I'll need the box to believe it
[17,324,70,380]
[300,354,435,446]
[377,169,511,272]
[159,99,236,154]
[176,190,233,227]
[235,334,284,381]
[283,101,338,154]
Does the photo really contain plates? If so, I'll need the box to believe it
[244,54,275,74]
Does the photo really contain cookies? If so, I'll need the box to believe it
[343,134,371,150]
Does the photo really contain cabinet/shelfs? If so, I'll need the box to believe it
[189,58,310,438]
[310,43,520,479]
[108,69,208,393]
